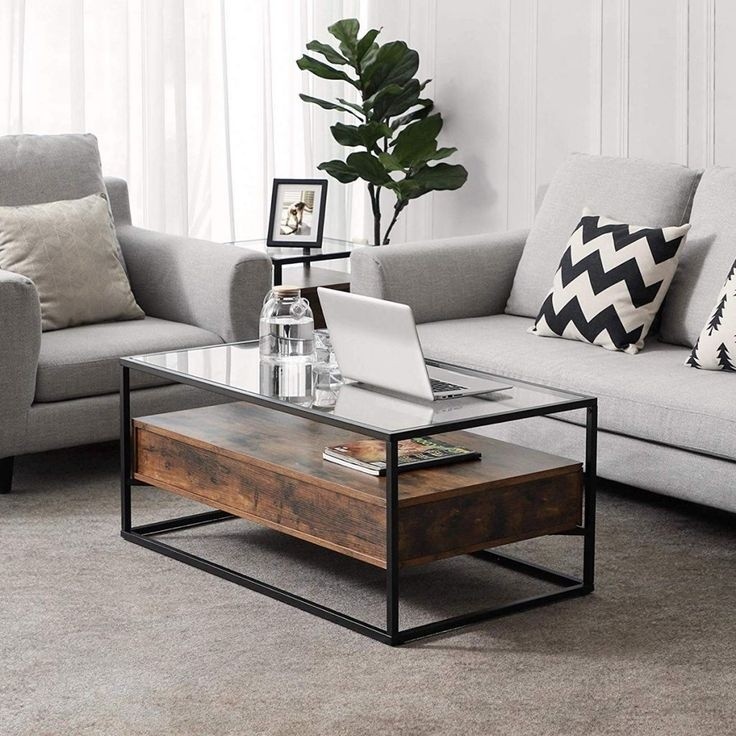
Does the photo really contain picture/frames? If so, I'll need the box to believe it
[265,179,328,248]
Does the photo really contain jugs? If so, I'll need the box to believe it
[260,361,313,408]
[259,285,315,363]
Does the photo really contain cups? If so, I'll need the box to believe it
[312,370,345,409]
[311,328,339,371]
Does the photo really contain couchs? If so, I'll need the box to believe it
[0,133,273,495]
[350,152,736,514]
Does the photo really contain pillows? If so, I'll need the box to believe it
[527,206,692,356]
[684,255,736,373]
[0,194,146,333]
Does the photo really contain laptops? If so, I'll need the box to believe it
[317,287,512,402]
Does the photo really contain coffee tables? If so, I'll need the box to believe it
[119,341,596,647]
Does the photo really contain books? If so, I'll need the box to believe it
[325,439,481,476]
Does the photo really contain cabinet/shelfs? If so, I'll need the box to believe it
[225,239,369,331]
